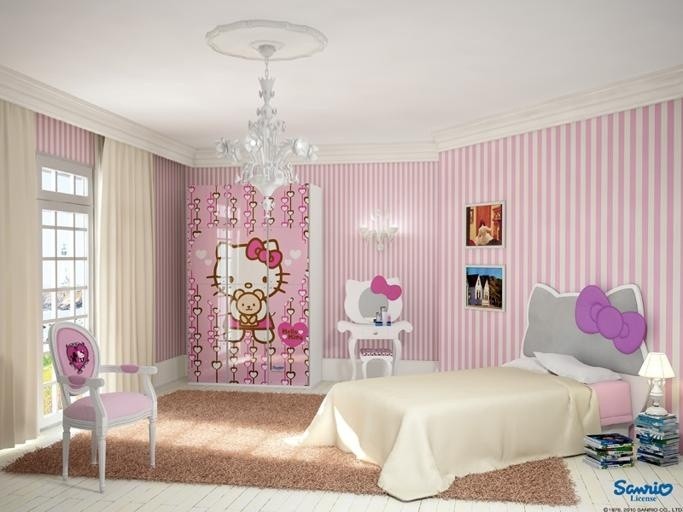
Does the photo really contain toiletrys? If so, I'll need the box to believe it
[374,306,392,327]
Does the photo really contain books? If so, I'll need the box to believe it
[580,409,680,470]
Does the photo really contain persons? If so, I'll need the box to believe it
[476,218,494,246]
